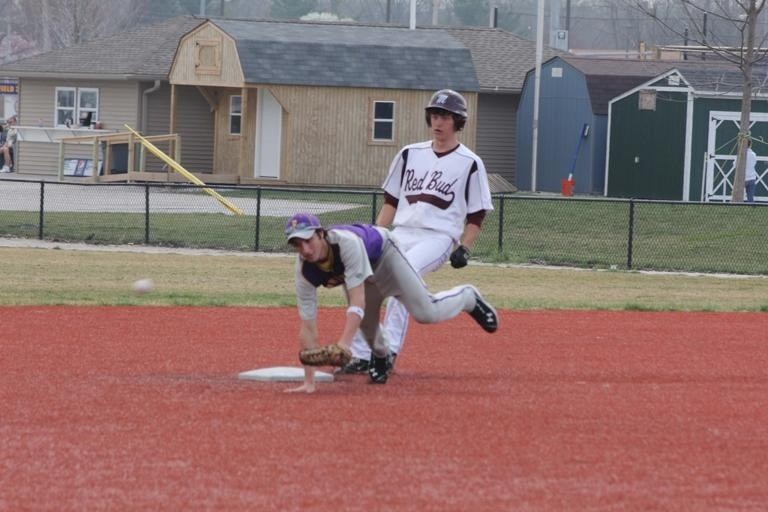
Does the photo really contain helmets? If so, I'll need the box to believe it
[424,90,467,132]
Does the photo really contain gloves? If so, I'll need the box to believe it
[450,246,470,268]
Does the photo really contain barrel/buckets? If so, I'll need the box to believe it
[561,179,574,195]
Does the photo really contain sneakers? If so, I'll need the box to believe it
[345,354,394,384]
[0,164,13,173]
[463,283,498,333]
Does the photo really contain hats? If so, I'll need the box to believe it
[284,214,323,245]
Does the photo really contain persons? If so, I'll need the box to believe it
[330,89,495,374]
[0,116,19,174]
[733,138,757,202]
[283,214,499,395]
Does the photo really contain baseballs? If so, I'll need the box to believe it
[132,279,153,297]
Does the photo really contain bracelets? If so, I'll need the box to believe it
[346,306,364,323]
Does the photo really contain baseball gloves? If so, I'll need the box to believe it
[299,344,352,367]
